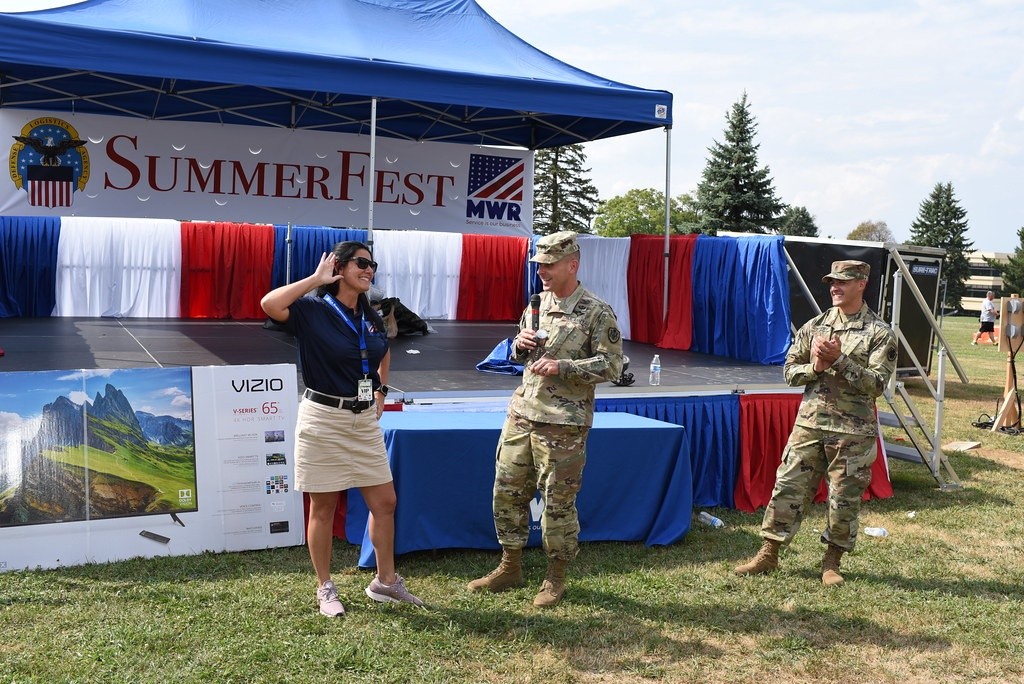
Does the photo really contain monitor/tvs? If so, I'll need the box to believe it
[0,366,199,527]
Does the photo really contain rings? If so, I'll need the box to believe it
[542,368,545,371]
[819,350,822,355]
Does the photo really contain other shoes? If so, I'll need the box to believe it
[971,340,978,345]
[993,343,998,346]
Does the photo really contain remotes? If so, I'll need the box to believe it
[139,530,170,544]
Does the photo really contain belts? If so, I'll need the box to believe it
[304,389,374,414]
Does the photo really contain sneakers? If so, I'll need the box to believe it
[365,573,423,608]
[317,580,345,617]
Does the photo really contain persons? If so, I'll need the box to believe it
[467,231,625,609]
[261,241,425,618]
[971,291,999,346]
[733,261,897,587]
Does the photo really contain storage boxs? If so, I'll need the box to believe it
[717,231,948,375]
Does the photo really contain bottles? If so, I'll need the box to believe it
[649,354,661,385]
[698,511,725,528]
[863,527,888,537]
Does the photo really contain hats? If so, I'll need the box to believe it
[822,260,871,282]
[529,231,580,264]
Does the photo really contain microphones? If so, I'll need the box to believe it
[531,294,541,333]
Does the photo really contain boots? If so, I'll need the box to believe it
[735,538,781,576]
[821,545,844,587]
[468,548,523,593]
[533,559,566,609]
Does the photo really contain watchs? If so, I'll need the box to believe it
[376,384,389,397]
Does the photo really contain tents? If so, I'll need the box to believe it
[0,0,673,338]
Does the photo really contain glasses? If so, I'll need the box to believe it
[344,257,378,274]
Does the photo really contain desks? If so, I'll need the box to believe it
[346,410,691,570]
